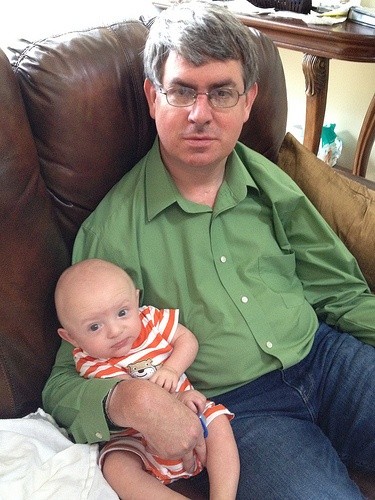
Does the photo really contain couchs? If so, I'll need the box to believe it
[1,13,375,500]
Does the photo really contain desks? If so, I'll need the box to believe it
[153,2,375,180]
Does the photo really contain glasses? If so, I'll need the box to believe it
[158,86,246,108]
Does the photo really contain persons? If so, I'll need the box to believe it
[53,256,243,500]
[38,0,375,499]
